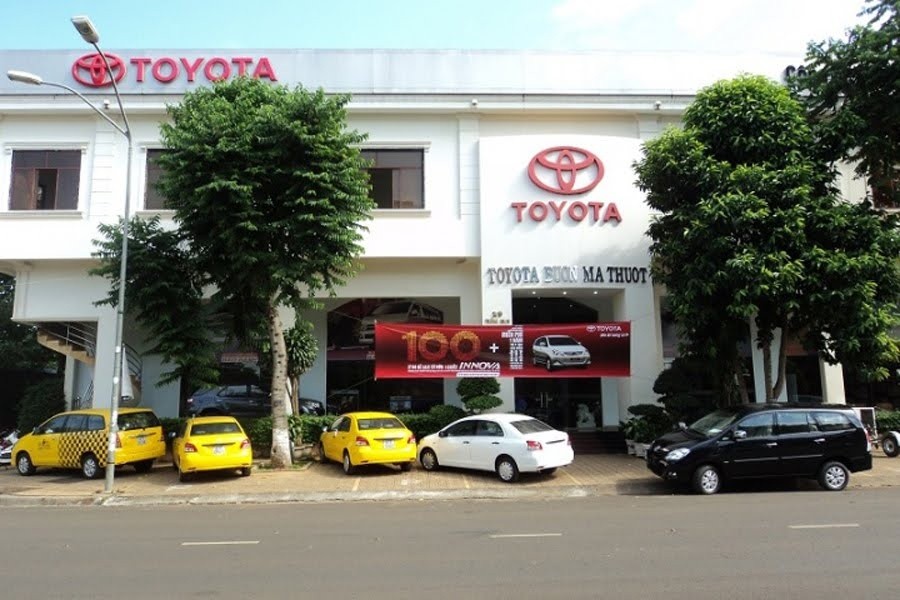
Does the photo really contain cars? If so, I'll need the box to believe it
[311,411,417,474]
[169,415,253,482]
[533,335,591,371]
[185,381,325,419]
[418,413,575,483]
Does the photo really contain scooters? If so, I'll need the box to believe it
[0,424,23,470]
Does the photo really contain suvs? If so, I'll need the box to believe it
[646,402,871,494]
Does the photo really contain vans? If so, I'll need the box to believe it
[11,407,166,479]
[359,300,441,345]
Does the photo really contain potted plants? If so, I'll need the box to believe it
[618,414,662,460]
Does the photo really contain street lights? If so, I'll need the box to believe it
[6,14,136,496]
